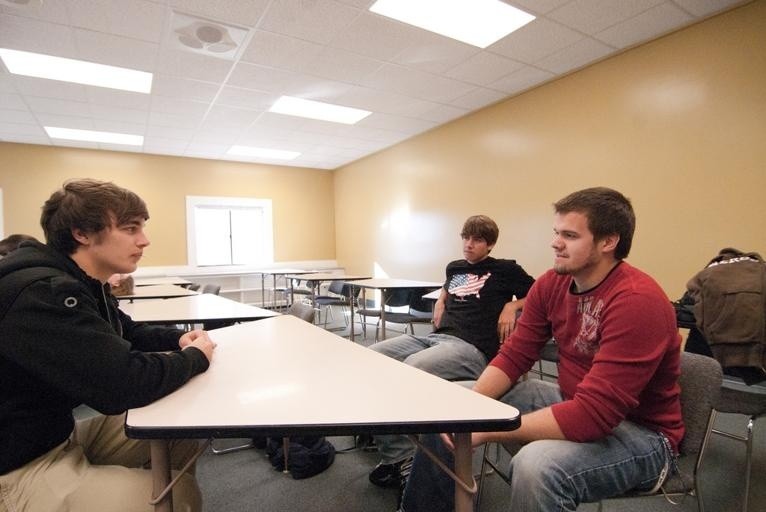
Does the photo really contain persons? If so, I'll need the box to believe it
[0,175,217,512]
[437,185,685,512]
[354,214,539,488]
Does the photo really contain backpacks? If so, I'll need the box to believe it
[252,435,339,481]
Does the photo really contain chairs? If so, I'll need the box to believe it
[471,338,765,512]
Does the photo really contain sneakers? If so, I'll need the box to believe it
[367,456,413,484]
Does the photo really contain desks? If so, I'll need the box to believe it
[112,267,524,505]
